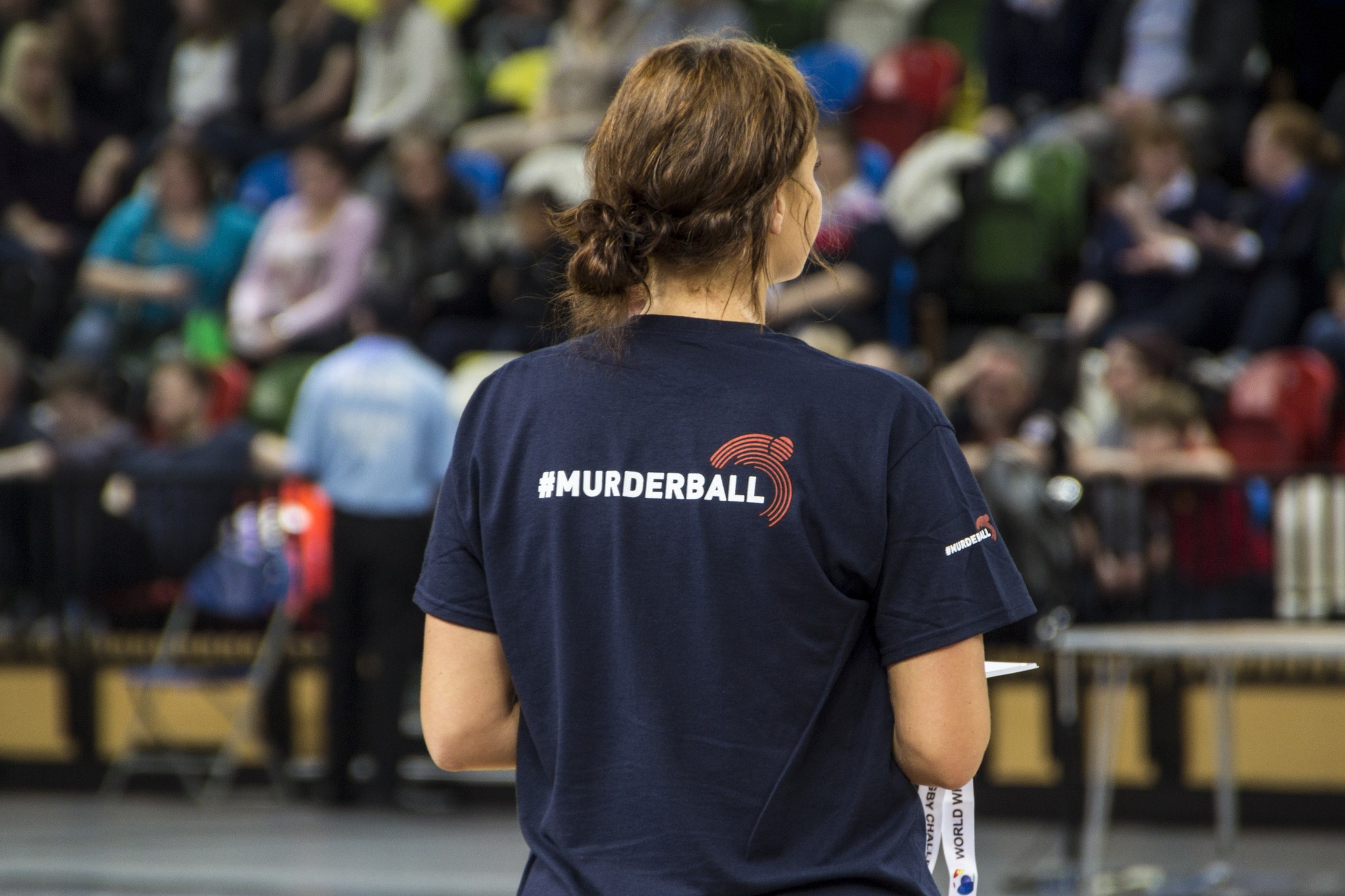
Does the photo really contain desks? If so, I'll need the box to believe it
[1058,616,1345,896]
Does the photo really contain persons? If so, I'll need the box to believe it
[1,0,1344,808]
[411,33,1039,896]
